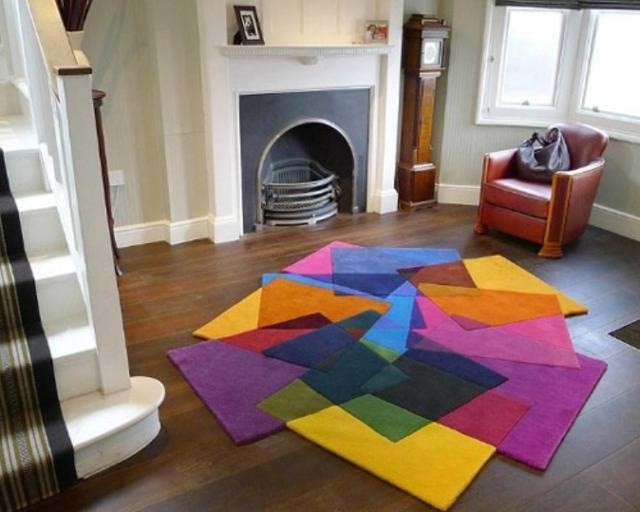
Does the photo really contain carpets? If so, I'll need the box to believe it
[166,240,608,512]
[608,312,640,354]
[2,148,79,511]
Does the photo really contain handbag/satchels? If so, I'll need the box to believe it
[515,128,570,181]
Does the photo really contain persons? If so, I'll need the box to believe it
[244,16,257,36]
[365,24,383,40]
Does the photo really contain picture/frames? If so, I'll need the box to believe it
[233,6,265,45]
[363,19,389,44]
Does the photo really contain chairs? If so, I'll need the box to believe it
[473,121,609,258]
